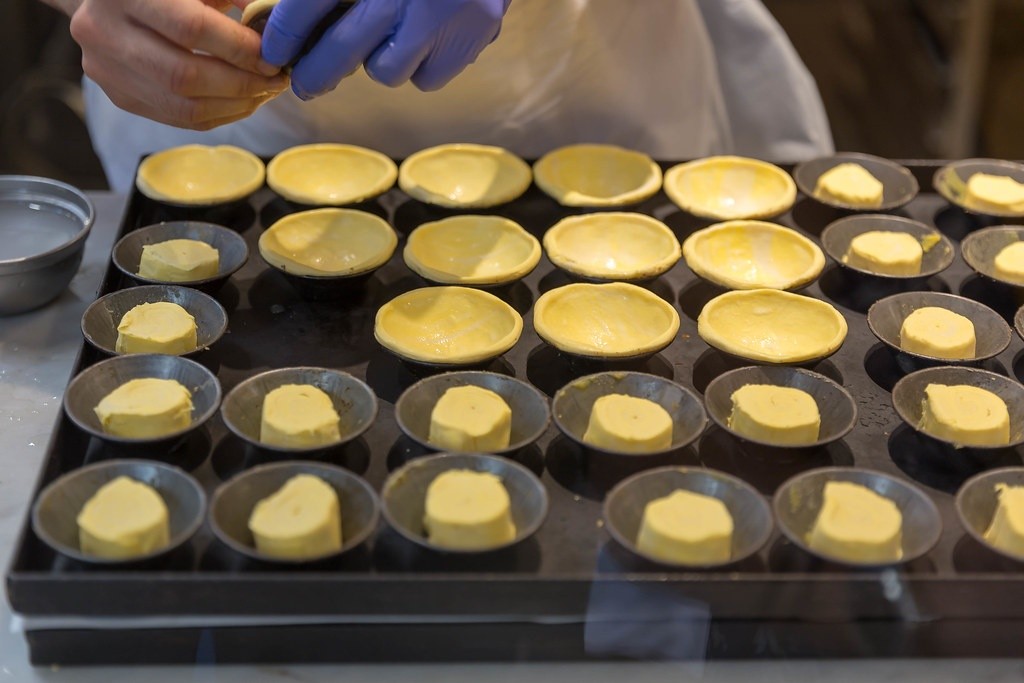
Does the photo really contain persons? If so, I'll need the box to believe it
[41,0,837,197]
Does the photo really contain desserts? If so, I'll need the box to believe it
[77,142,1024,562]
[241,0,275,29]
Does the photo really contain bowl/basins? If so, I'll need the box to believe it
[774,467,943,569]
[31,458,207,568]
[380,453,547,559]
[246,8,326,76]
[604,465,773,569]
[210,461,379,566]
[954,467,1024,570]
[0,174,95,314]
[62,151,1024,459]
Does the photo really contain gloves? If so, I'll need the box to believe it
[261,0,512,102]
